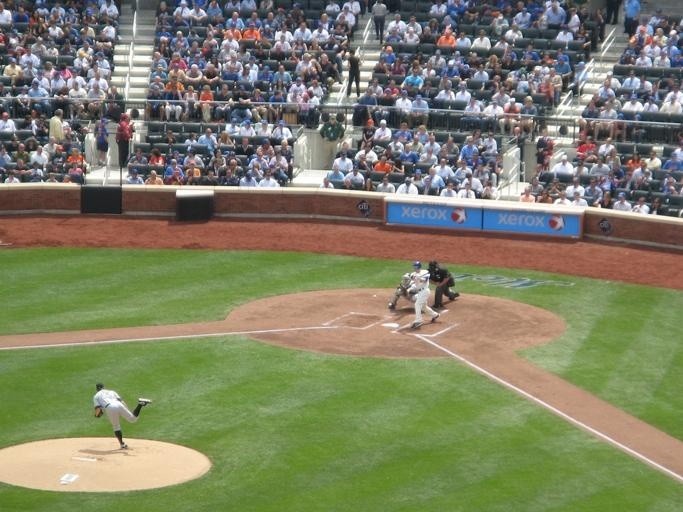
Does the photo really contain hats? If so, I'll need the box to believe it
[96,383,104,389]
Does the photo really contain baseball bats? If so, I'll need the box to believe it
[420,268,438,277]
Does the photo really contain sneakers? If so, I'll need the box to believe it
[138,398,152,406]
[431,313,440,323]
[411,322,423,330]
[449,293,459,299]
[431,303,443,308]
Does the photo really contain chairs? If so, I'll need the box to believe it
[0,0,683,232]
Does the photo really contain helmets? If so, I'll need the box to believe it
[412,261,421,268]
[429,260,438,271]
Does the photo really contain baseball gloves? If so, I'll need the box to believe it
[396,284,407,296]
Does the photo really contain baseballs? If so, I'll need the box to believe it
[373,295,377,298]
[399,288,401,292]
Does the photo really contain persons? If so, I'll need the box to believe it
[388,272,418,308]
[427,260,460,308]
[410,260,440,328]
[93,382,153,448]
